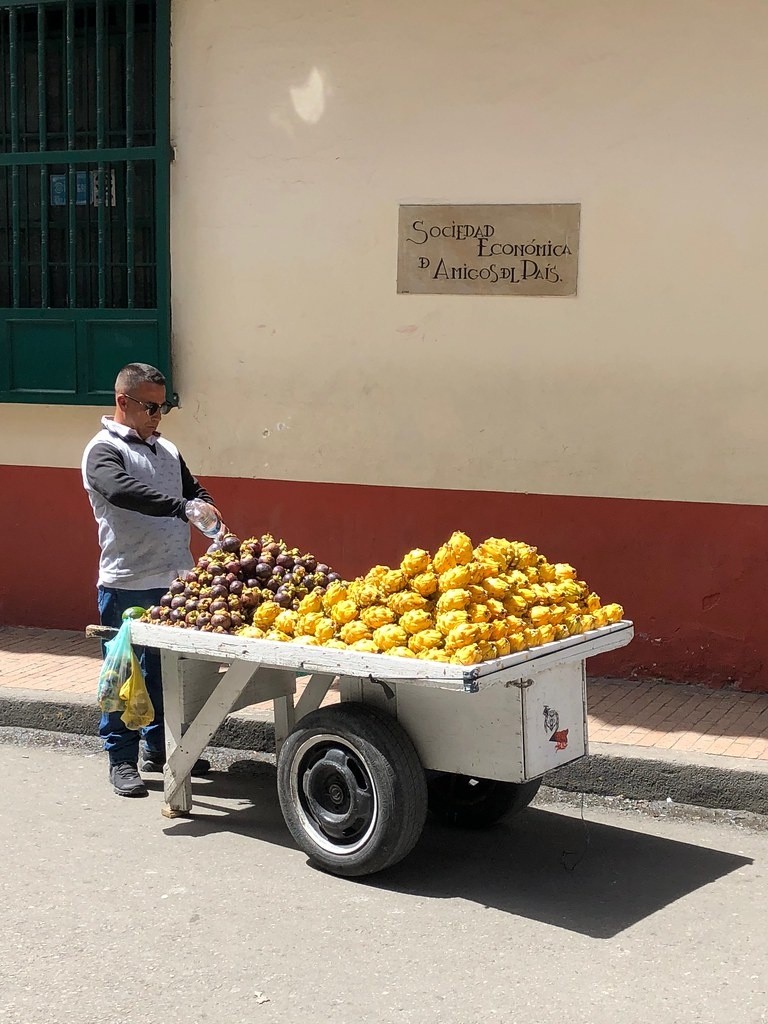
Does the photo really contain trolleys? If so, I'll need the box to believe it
[85,616,635,876]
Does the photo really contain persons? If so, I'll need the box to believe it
[83,362,223,797]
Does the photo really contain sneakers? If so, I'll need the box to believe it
[141,749,210,774]
[109,762,147,795]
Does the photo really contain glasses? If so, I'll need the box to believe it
[120,393,173,416]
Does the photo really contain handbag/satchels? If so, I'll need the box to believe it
[98,618,154,730]
